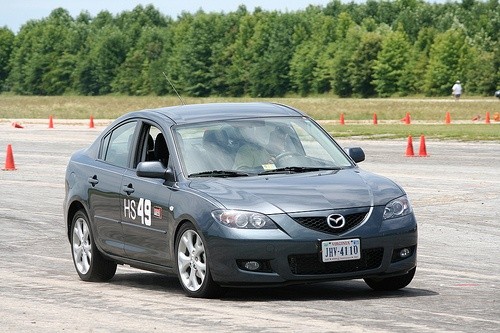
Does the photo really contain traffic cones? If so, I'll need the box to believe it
[486,112,490,123]
[47,115,54,128]
[88,114,95,128]
[405,112,411,124]
[493,113,500,121]
[446,112,451,124]
[14,122,24,128]
[400,117,406,121]
[340,111,345,124]
[402,135,417,157]
[372,112,378,124]
[1,143,17,171]
[417,134,430,157]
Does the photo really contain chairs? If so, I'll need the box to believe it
[215,125,245,166]
[148,132,209,168]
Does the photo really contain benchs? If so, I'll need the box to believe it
[124,128,235,168]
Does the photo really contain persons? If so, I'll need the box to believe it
[452,79,462,102]
[231,124,293,171]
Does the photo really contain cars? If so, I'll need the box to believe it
[63,102,418,300]
